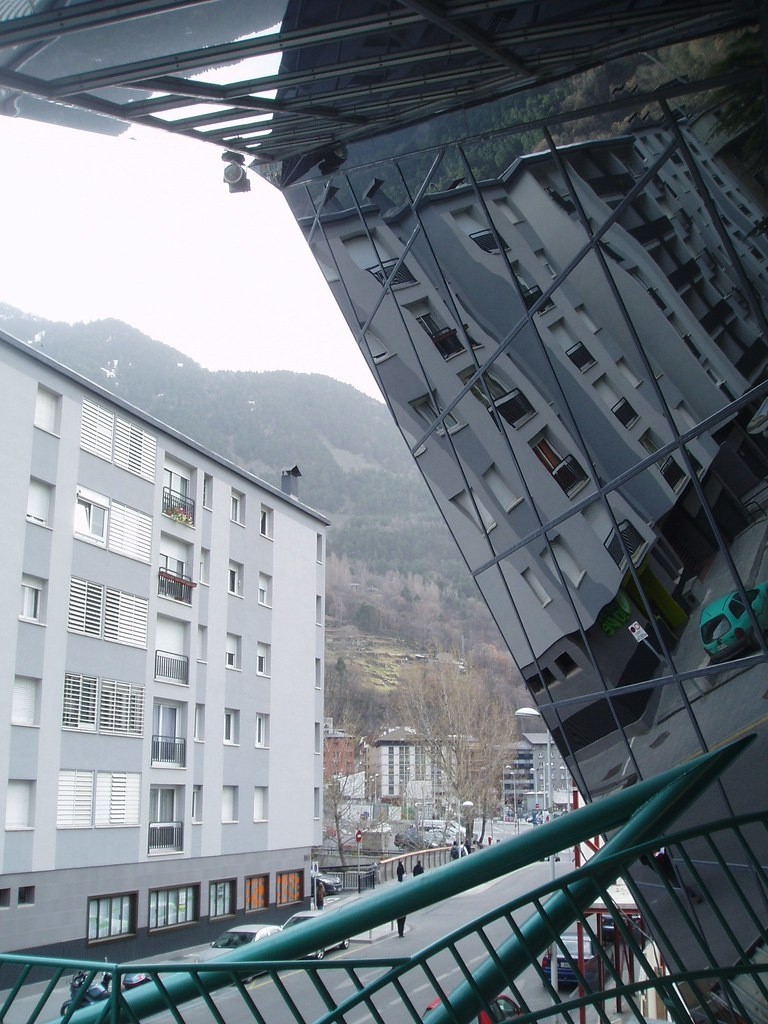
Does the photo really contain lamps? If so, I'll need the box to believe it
[221,151,251,194]
[318,146,349,176]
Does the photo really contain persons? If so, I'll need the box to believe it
[397,915,406,938]
[450,839,471,859]
[397,860,424,882]
[317,882,326,910]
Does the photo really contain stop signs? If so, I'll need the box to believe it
[535,803,540,809]
[356,831,362,843]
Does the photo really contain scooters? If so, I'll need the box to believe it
[59,955,154,1018]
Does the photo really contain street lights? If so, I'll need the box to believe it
[559,762,571,812]
[374,773,379,803]
[457,798,474,859]
[529,767,539,810]
[502,765,511,821]
[515,707,553,821]
[746,500,768,517]
[510,771,518,822]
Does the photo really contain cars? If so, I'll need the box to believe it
[311,870,343,898]
[541,935,614,986]
[393,820,480,852]
[698,578,768,663]
[602,914,642,943]
[282,910,350,960]
[421,991,529,1024]
[194,925,284,984]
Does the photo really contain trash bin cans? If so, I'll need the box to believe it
[682,576,707,608]
